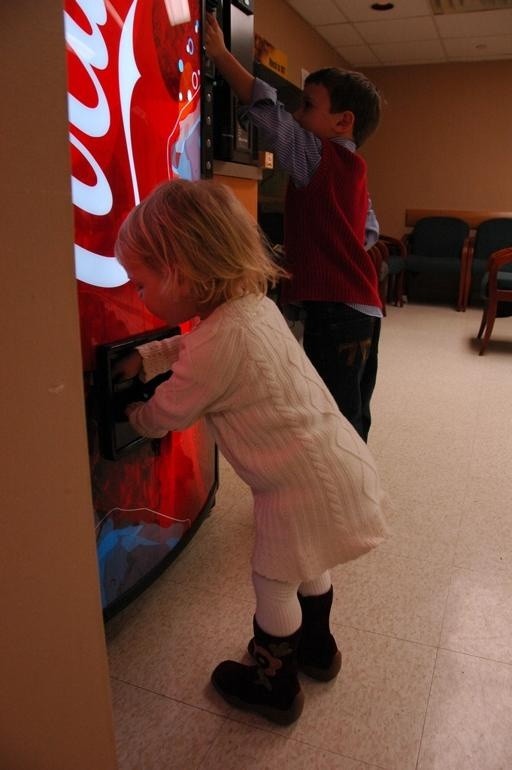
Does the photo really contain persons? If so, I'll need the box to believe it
[108,177,394,724]
[198,10,388,444]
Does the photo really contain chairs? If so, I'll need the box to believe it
[367,215,511,356]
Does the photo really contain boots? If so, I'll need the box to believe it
[245,582,343,685]
[210,613,307,728]
